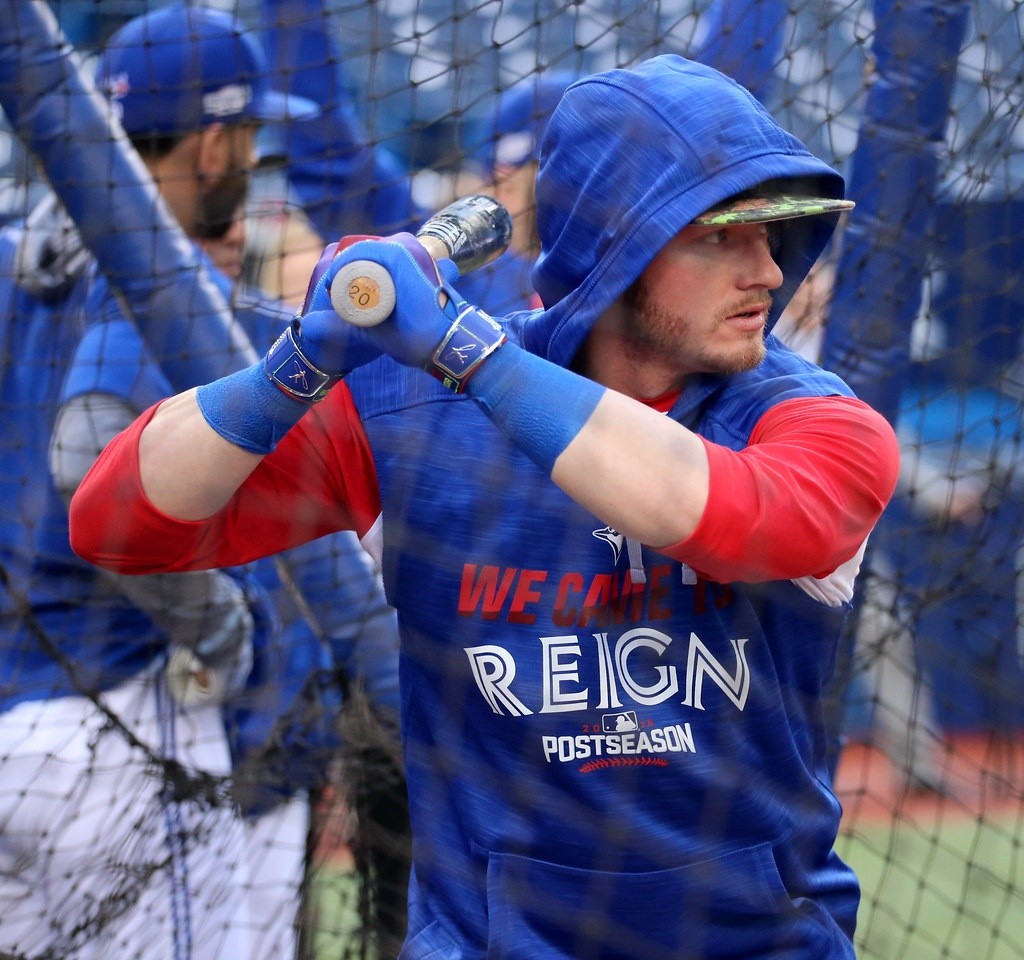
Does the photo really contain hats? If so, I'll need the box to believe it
[94,6,323,134]
[691,177,859,227]
[483,67,591,170]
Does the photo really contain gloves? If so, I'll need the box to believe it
[300,231,606,481]
[195,243,460,456]
[163,641,254,713]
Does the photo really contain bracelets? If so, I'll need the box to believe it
[428,301,509,394]
[262,325,345,404]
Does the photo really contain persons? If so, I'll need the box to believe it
[0,2,268,960]
[67,52,902,960]
[23,68,583,960]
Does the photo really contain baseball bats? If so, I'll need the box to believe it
[329,195,514,328]
